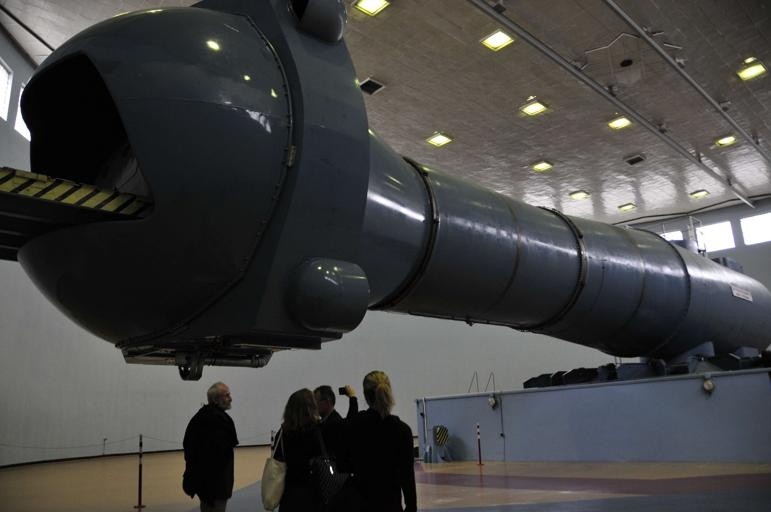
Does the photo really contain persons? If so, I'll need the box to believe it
[181,381,239,512]
[313,385,343,425]
[335,370,418,512]
[272,383,359,512]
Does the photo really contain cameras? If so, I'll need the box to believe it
[338,386,346,395]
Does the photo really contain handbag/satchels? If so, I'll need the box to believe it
[309,453,352,505]
[260,424,288,512]
